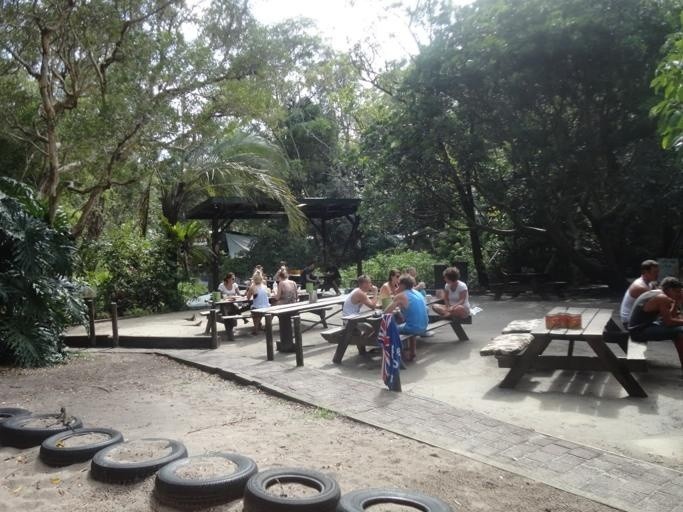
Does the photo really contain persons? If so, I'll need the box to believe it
[300,264,321,289]
[244,259,299,332]
[627,275,683,365]
[379,266,435,362]
[218,272,249,332]
[432,266,471,322]
[323,260,341,296]
[620,259,665,332]
[342,275,379,355]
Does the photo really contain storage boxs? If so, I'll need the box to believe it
[480,318,539,368]
[626,336,649,371]
[197,306,474,371]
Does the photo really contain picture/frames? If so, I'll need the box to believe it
[496,306,648,396]
[204,290,444,369]
